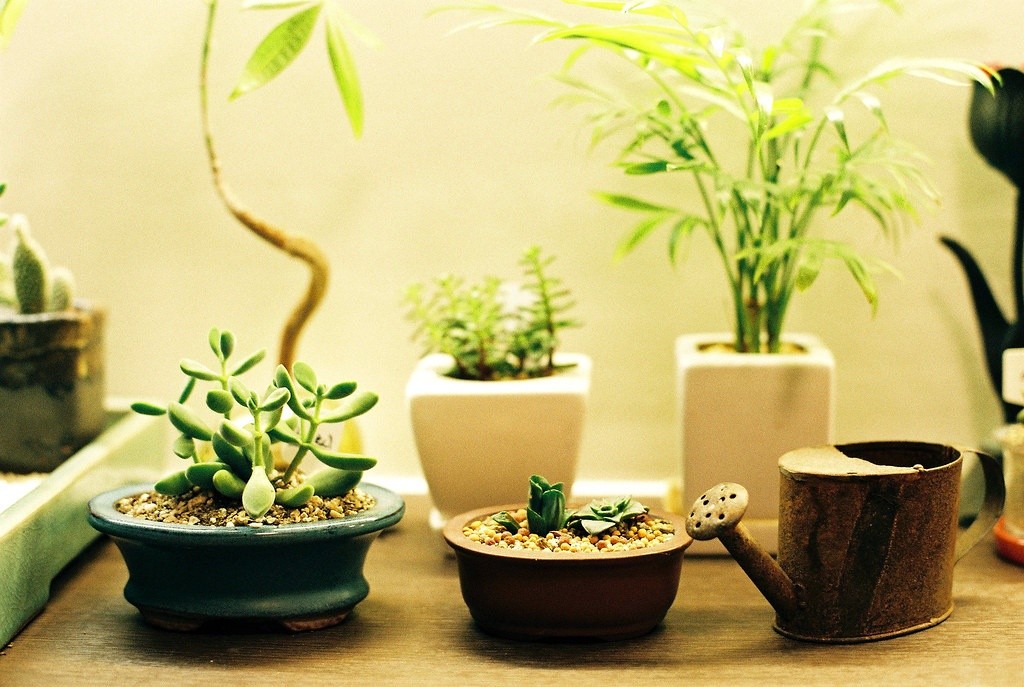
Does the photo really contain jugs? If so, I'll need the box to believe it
[685,440,1007,645]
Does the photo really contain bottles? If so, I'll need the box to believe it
[993,346,1024,566]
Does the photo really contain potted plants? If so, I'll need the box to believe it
[0,183,110,470]
[400,251,592,524]
[440,472,697,640]
[89,4,407,644]
[426,1,1005,513]
